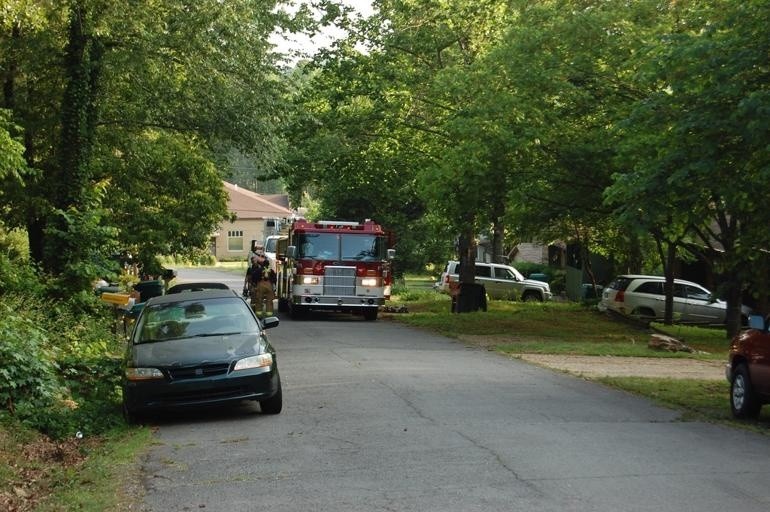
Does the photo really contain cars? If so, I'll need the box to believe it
[721,308,769,421]
[118,285,286,426]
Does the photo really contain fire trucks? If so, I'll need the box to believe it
[275,213,398,324]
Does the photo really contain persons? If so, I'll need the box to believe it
[243,255,277,320]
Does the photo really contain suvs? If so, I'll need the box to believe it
[430,259,553,304]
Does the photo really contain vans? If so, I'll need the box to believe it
[600,272,754,329]
[263,234,288,275]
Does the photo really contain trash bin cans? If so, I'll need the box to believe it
[530,273,546,282]
[134,280,163,303]
